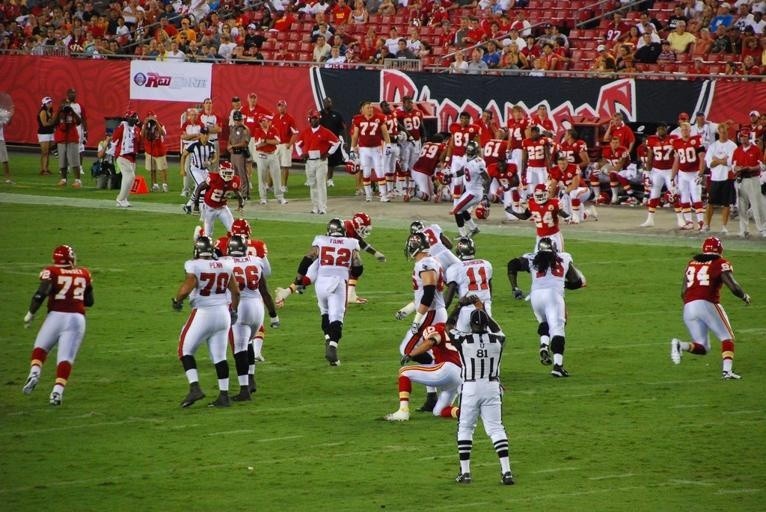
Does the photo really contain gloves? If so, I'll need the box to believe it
[400,354,411,366]
[457,293,475,307]
[270,317,281,329]
[293,278,307,294]
[512,286,526,301]
[23,311,34,331]
[375,251,387,264]
[742,293,752,305]
[171,296,184,311]
[410,322,421,336]
[395,311,407,321]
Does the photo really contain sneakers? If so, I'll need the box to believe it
[670,338,682,364]
[551,364,569,377]
[249,179,334,213]
[42,166,206,213]
[721,370,741,380]
[232,382,257,402]
[49,392,62,405]
[253,353,265,362]
[355,190,766,241]
[208,395,230,408]
[384,408,410,422]
[180,388,206,408]
[499,471,514,486]
[348,296,368,305]
[22,373,39,396]
[324,338,341,366]
[274,286,286,310]
[415,399,438,413]
[539,343,552,366]
[455,472,471,484]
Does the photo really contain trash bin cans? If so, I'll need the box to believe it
[104,117,125,141]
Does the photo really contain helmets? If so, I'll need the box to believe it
[230,219,252,239]
[410,221,424,233]
[326,218,345,236]
[703,237,723,256]
[537,237,554,253]
[456,237,475,258]
[228,235,246,256]
[346,160,361,174]
[352,213,372,238]
[194,237,214,257]
[405,232,429,258]
[219,161,234,180]
[53,244,75,267]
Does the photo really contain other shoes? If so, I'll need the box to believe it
[6,177,11,185]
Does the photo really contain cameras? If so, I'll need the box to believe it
[148,120,156,127]
[62,105,71,113]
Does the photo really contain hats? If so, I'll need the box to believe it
[543,23,552,29]
[247,23,256,30]
[719,2,731,10]
[181,18,190,25]
[469,310,490,326]
[40,94,761,152]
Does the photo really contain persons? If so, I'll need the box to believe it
[1,89,765,241]
[671,235,752,379]
[20,245,94,405]
[1,0,765,79]
[173,212,387,409]
[446,293,514,483]
[386,221,588,423]
